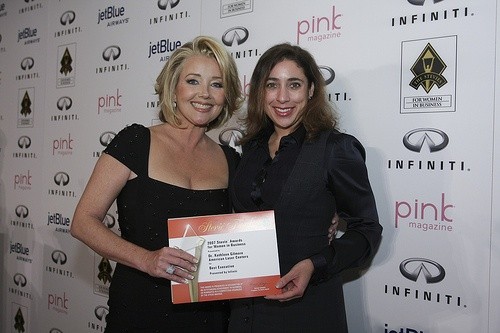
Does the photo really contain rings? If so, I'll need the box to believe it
[165,265,176,274]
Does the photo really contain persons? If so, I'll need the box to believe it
[229,43,382,333]
[70,35,338,333]
